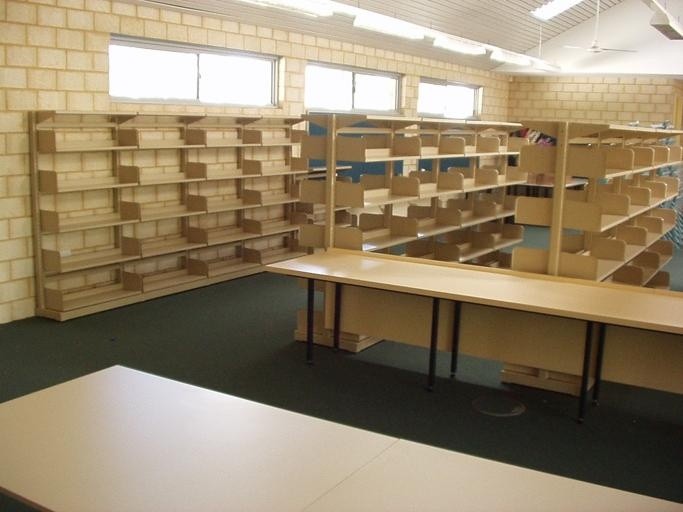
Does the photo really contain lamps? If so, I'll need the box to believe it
[650,13,682,40]
[280,0,565,74]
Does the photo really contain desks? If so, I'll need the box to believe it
[506,178,587,196]
[0,365,399,512]
[427,265,603,423]
[578,283,681,424]
[294,437,681,512]
[263,246,460,391]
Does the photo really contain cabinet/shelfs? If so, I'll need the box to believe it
[293,113,526,353]
[29,108,307,321]
[499,118,682,397]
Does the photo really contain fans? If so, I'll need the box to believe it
[562,0,636,54]
